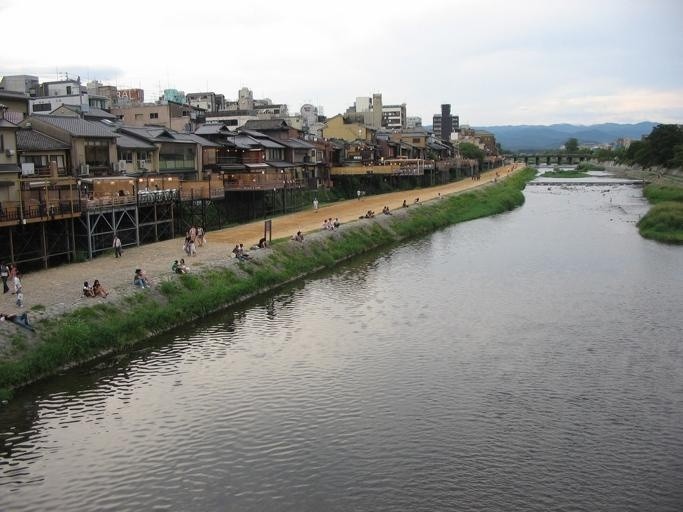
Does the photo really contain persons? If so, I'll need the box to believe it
[238,244,254,260]
[357,189,422,219]
[134,269,151,289]
[293,231,304,242]
[83,282,96,296]
[323,216,341,232]
[113,234,123,258]
[172,223,207,276]
[93,280,109,298]
[258,237,267,249]
[313,197,319,210]
[0,260,35,333]
[232,244,249,261]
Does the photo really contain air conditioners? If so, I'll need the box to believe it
[114,160,127,172]
[79,165,90,176]
[138,160,146,169]
